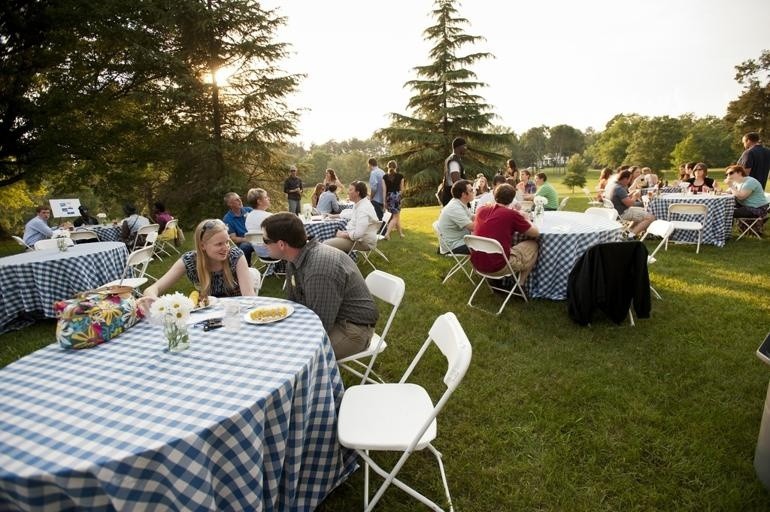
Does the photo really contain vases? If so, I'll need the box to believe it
[57,239,65,253]
[304,211,312,222]
[533,206,544,222]
[164,323,189,350]
[682,188,688,197]
[99,220,105,226]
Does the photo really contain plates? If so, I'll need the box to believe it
[192,295,218,315]
[246,304,294,326]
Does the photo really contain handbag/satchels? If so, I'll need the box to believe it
[54,284,154,350]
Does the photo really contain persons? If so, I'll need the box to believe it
[477,175,518,212]
[605,165,629,186]
[444,137,472,209]
[474,178,491,195]
[724,166,768,218]
[679,164,689,181]
[223,192,252,265]
[321,181,379,252]
[245,189,282,261]
[474,174,484,183]
[74,206,99,244]
[369,158,388,236]
[471,183,539,299]
[317,185,339,215]
[741,132,770,192]
[311,183,325,209]
[524,173,558,211]
[515,170,536,194]
[688,164,717,192]
[260,211,379,360]
[122,205,153,247]
[383,160,405,241]
[23,205,53,252]
[629,167,657,192]
[439,180,476,254]
[504,159,519,183]
[284,166,302,214]
[597,168,613,202]
[610,170,656,239]
[135,219,254,310]
[686,162,695,179]
[152,202,172,235]
[630,166,640,182]
[324,168,344,194]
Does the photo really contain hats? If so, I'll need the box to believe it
[452,137,466,148]
[123,203,137,214]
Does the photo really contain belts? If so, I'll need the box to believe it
[352,322,377,328]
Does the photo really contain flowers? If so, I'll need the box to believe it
[301,203,313,219]
[97,213,107,224]
[679,181,690,196]
[534,196,548,217]
[151,291,196,347]
[50,229,71,250]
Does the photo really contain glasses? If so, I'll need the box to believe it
[200,220,216,240]
[262,236,273,246]
[725,171,734,176]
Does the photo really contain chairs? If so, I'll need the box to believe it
[630,219,673,301]
[664,204,707,255]
[567,241,648,332]
[337,271,406,386]
[129,224,163,265]
[32,238,57,252]
[243,231,293,290]
[463,235,529,317]
[337,311,477,512]
[249,269,261,295]
[11,235,33,252]
[602,199,635,239]
[432,221,478,286]
[156,219,180,257]
[345,221,391,271]
[584,206,618,223]
[96,245,154,297]
[125,231,159,282]
[582,188,601,207]
[70,230,100,244]
[734,192,770,245]
[362,211,393,264]
[555,196,570,211]
